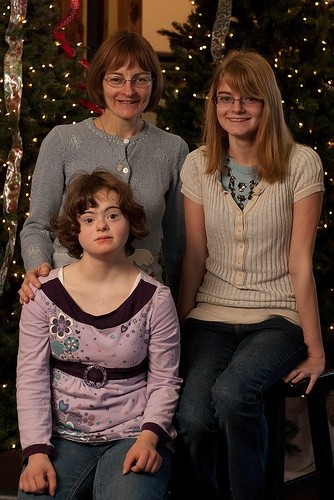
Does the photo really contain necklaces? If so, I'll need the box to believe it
[223,149,268,210]
[98,114,143,173]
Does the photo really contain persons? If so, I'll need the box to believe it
[16,31,193,322]
[16,166,179,499]
[168,51,325,500]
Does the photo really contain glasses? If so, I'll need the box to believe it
[214,95,264,106]
[102,75,155,87]
